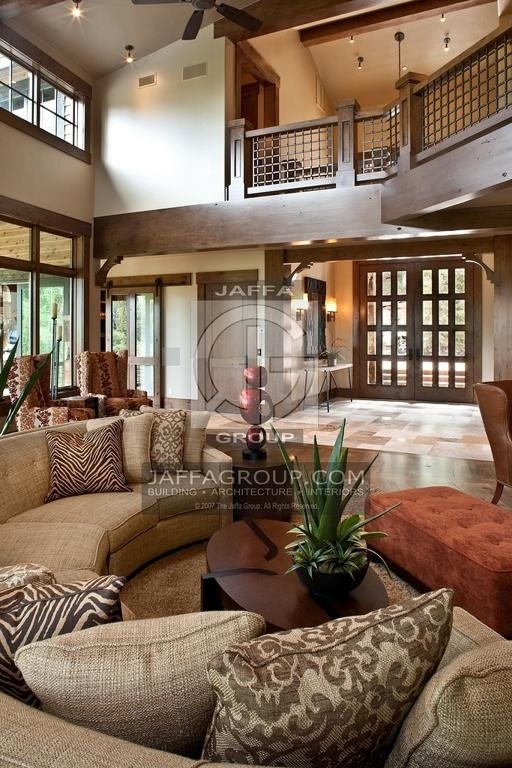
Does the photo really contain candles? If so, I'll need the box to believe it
[51,303,57,318]
[56,325,62,341]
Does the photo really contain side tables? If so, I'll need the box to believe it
[61,396,99,417]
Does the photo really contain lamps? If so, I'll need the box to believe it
[444,38,450,51]
[71,0,82,17]
[358,57,363,69]
[292,294,309,321]
[327,298,337,321]
[125,46,134,62]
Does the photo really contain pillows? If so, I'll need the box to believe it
[45,404,211,503]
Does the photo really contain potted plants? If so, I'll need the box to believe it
[319,336,350,367]
[269,420,405,601]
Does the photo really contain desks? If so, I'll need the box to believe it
[227,448,295,517]
[318,363,354,412]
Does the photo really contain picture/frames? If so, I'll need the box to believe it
[304,277,327,359]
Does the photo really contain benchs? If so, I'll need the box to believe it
[363,486,512,636]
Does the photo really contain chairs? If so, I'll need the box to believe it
[72,350,153,412]
[474,380,512,505]
[6,351,95,432]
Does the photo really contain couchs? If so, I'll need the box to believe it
[0,423,512,767]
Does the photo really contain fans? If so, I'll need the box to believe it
[133,0,263,40]
